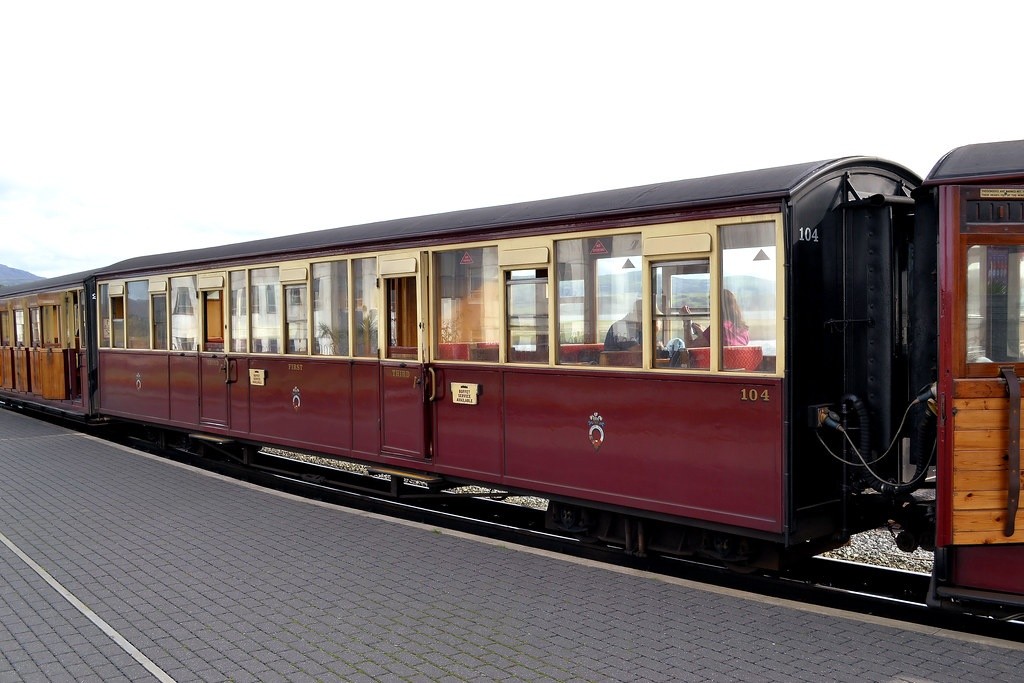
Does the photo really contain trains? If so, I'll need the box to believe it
[0,136,1024,646]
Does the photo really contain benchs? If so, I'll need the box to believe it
[469,344,549,362]
[388,346,418,360]
[439,342,499,360]
[600,351,643,368]
[681,345,764,371]
[560,343,605,363]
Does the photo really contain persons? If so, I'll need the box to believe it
[680,289,750,349]
[604,299,664,350]
